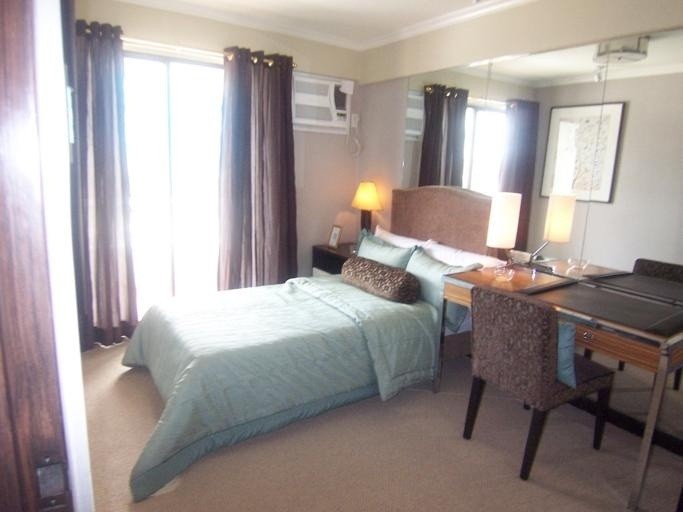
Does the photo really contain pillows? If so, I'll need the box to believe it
[341,225,507,334]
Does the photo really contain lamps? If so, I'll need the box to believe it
[485,191,522,269]
[350,181,383,231]
[530,193,577,263]
[593,36,650,65]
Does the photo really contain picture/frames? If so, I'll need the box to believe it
[326,224,343,250]
[538,101,624,204]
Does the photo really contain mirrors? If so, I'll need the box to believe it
[397,28,683,442]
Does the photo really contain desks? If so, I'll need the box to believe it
[432,265,682,512]
[511,257,683,306]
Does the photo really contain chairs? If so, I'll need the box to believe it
[583,258,682,390]
[461,282,615,480]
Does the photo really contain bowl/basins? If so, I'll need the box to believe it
[567,257,591,271]
[494,268,515,282]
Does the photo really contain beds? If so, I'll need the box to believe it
[151,184,491,451]
